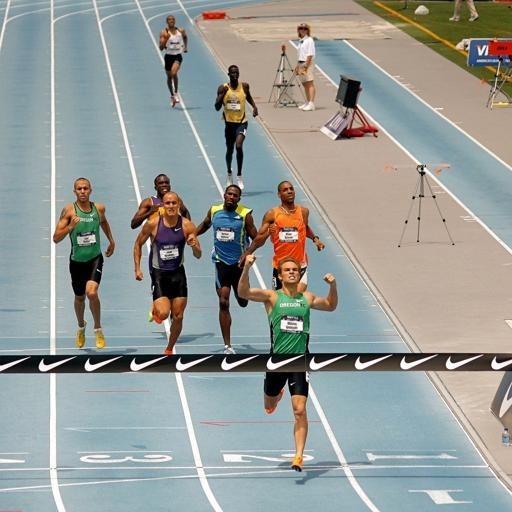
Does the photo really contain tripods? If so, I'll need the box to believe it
[268,54,307,108]
[398,165,455,247]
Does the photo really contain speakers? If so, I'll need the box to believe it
[335,75,360,108]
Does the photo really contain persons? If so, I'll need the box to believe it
[237,254,339,473]
[237,181,325,293]
[133,193,201,356]
[295,23,316,111]
[131,173,191,229]
[195,185,258,354]
[215,64,259,191]
[53,177,115,350]
[159,15,188,107]
[448,0,479,22]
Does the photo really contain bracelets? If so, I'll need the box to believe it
[312,236,319,243]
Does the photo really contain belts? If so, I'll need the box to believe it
[298,60,305,63]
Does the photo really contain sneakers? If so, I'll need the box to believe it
[148,304,173,355]
[266,389,284,413]
[94,328,105,349]
[224,345,235,354]
[76,320,87,347]
[237,176,244,190]
[291,457,302,472]
[227,168,233,187]
[298,102,315,111]
[448,14,480,21]
[170,93,179,108]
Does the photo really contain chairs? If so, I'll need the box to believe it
[273,66,298,108]
